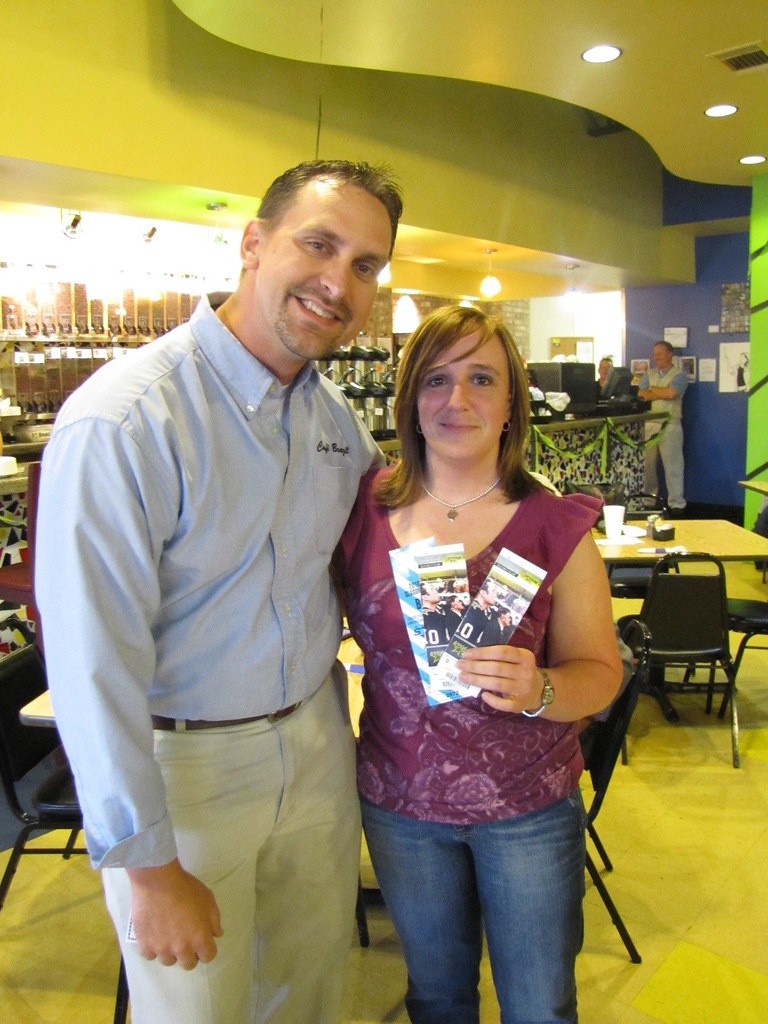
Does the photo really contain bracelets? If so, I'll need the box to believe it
[649,389,652,392]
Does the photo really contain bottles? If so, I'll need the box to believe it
[645,515,661,538]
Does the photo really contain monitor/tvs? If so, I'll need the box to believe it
[600,367,634,396]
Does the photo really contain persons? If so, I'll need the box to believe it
[328,305,624,1024]
[637,341,688,516]
[457,579,517,648]
[419,582,464,645]
[596,357,614,391]
[34,160,405,1024]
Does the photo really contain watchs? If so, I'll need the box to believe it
[521,668,555,718]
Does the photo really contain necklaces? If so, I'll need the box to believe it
[421,475,501,521]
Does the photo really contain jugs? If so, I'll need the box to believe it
[323,368,397,439]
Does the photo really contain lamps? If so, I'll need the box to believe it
[60,208,83,240]
[561,263,585,310]
[143,226,157,243]
[480,248,501,297]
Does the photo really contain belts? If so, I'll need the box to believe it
[152,701,303,731]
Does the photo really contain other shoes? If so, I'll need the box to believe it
[672,506,687,515]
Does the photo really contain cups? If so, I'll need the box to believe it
[603,505,625,540]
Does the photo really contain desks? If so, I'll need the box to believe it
[588,519,768,692]
[18,616,366,742]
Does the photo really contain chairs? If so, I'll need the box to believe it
[615,550,740,768]
[605,493,680,599]
[682,598,768,719]
[350,614,642,962]
[0,462,44,655]
[0,645,130,1024]
